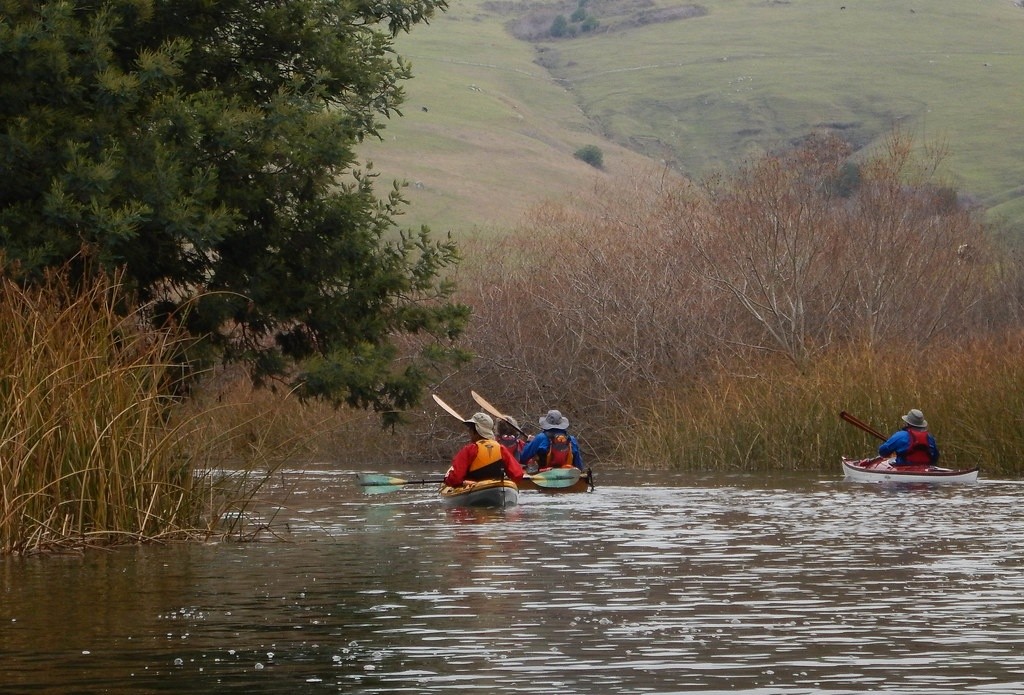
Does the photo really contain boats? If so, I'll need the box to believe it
[840,454,981,485]
[437,478,519,509]
[516,469,590,494]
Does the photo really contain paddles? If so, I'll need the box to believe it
[353,472,454,495]
[839,411,891,443]
[469,387,535,440]
[431,392,469,425]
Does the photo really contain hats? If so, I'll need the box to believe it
[462,413,495,439]
[538,410,569,430]
[494,415,518,436]
[901,409,927,427]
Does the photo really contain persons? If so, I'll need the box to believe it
[520,409,584,472]
[495,415,524,457]
[444,412,524,487]
[879,409,939,465]
